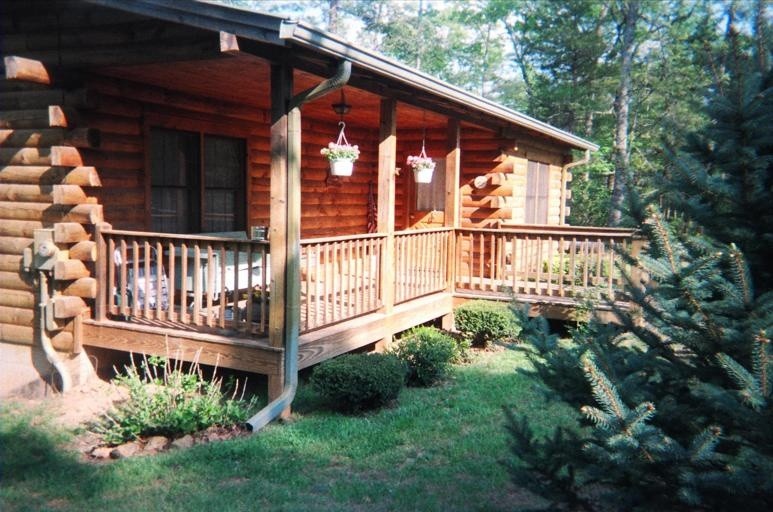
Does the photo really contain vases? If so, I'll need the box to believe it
[413,169,433,183]
[329,158,353,176]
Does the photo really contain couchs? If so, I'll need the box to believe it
[165,230,270,306]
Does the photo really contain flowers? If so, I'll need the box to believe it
[320,142,359,160]
[407,155,435,168]
